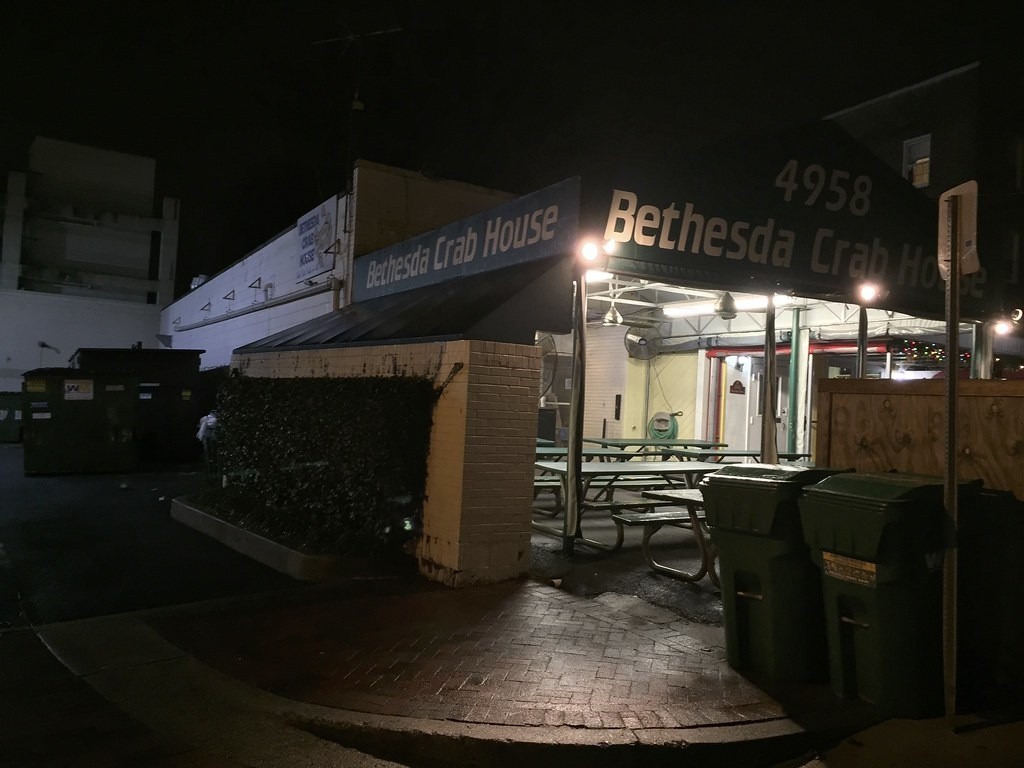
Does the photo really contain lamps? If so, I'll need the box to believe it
[1005,302,1023,320]
[715,290,739,321]
[602,301,624,328]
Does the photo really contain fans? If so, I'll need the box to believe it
[533,330,558,399]
[623,326,663,359]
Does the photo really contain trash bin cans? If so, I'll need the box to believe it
[695,464,986,717]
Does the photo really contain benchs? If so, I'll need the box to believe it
[534,459,861,591]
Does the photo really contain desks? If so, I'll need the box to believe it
[662,447,811,465]
[641,489,726,590]
[535,447,644,461]
[582,437,728,462]
[534,462,736,544]
[534,437,555,446]
